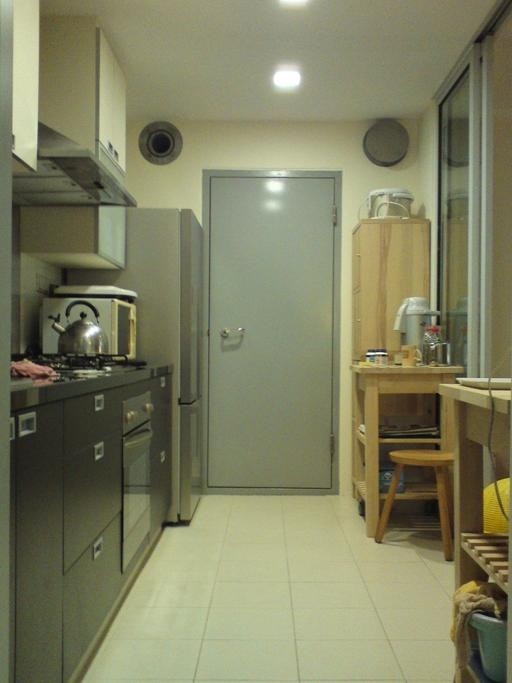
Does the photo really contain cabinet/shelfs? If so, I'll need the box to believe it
[11,373,173,680]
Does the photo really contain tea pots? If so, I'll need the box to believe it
[48,300,109,355]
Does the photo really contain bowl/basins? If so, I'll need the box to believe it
[467,612,506,683]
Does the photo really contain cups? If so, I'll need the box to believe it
[436,341,450,365]
[400,344,422,367]
[365,348,388,366]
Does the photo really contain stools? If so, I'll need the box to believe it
[374,448,456,563]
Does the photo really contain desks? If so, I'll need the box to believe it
[349,362,467,536]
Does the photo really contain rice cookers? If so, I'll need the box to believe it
[368,187,413,217]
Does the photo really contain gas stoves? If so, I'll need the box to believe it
[11,351,148,377]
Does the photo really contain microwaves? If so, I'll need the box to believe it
[37,297,136,361]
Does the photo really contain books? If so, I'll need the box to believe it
[358,420,440,438]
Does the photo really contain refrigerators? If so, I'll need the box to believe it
[65,207,208,527]
